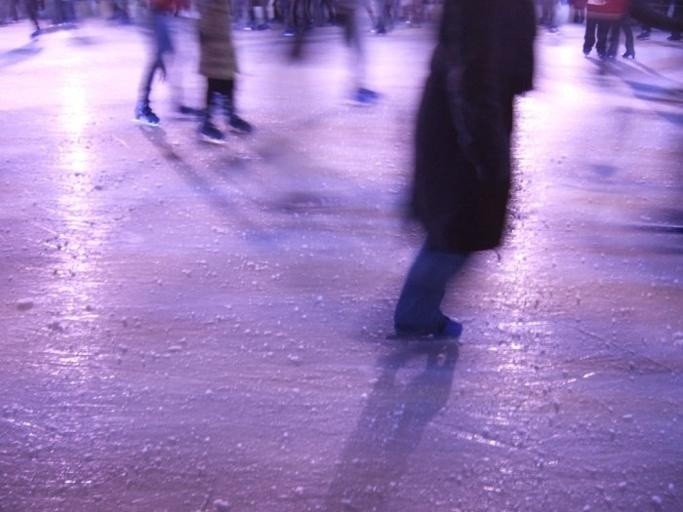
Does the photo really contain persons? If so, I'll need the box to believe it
[336,1,377,104]
[392,2,538,339]
[1,0,148,36]
[132,1,204,127]
[536,0,682,59]
[227,1,340,58]
[199,0,252,145]
[367,1,444,34]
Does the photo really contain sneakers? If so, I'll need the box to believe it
[133,102,158,124]
[227,114,250,131]
[636,32,648,37]
[583,41,634,57]
[201,123,224,139]
[178,105,203,115]
[394,309,461,337]
